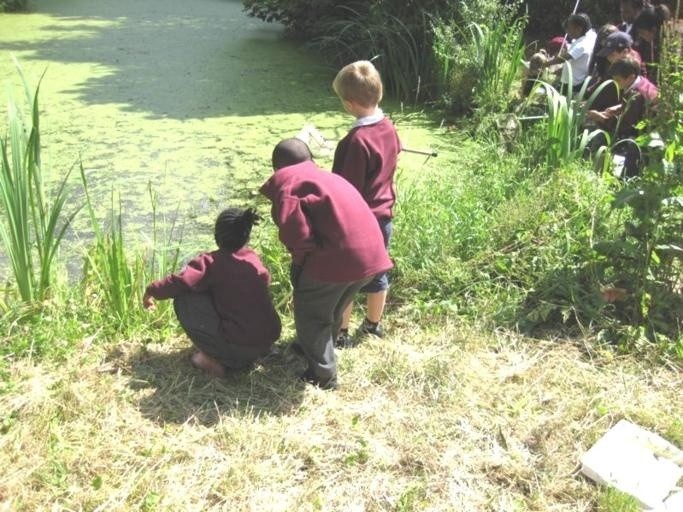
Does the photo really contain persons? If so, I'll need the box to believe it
[141,206,284,377]
[330,59,404,345]
[516,2,683,180]
[256,136,395,392]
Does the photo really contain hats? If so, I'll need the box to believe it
[596,31,633,57]
[549,37,568,47]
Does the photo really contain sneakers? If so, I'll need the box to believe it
[293,341,339,389]
